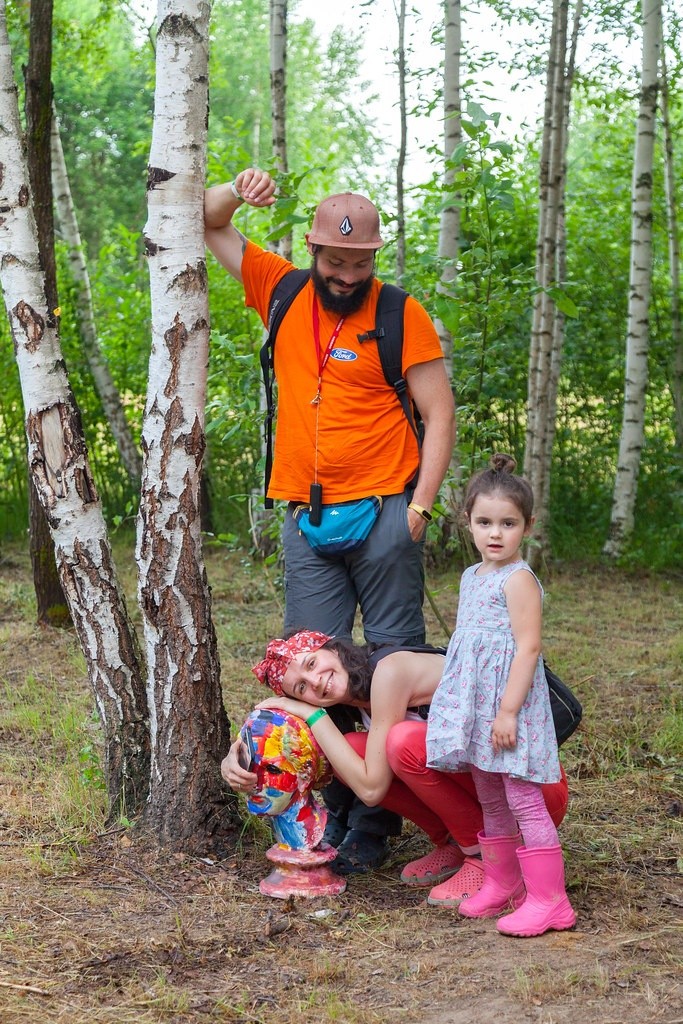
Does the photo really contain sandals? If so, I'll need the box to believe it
[329,829,392,875]
[400,845,464,885]
[319,822,346,847]
[428,856,486,906]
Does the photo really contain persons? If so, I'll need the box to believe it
[235,707,334,852]
[202,169,456,875]
[425,454,577,937]
[220,629,568,907]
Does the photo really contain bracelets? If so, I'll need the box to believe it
[231,180,244,201]
[305,707,327,728]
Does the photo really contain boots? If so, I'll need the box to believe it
[456,828,528,919]
[497,845,576,937]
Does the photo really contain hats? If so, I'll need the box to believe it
[308,191,384,249]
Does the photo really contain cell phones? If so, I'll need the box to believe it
[238,726,255,772]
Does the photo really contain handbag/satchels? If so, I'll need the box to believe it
[291,494,390,557]
[543,661,584,747]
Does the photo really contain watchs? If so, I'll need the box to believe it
[408,503,433,522]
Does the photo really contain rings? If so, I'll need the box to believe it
[237,784,241,790]
